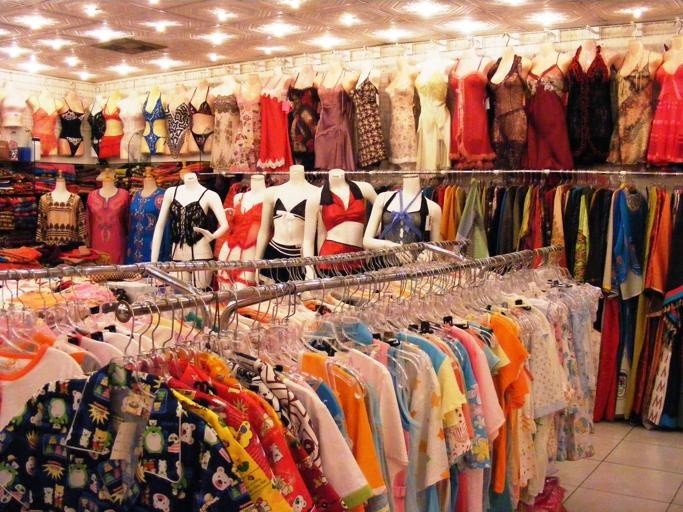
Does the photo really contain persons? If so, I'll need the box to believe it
[149,164,446,299]
[32,172,174,270]
[1,79,210,166]
[385,36,681,173]
[210,59,384,172]
[152,125,153,127]
